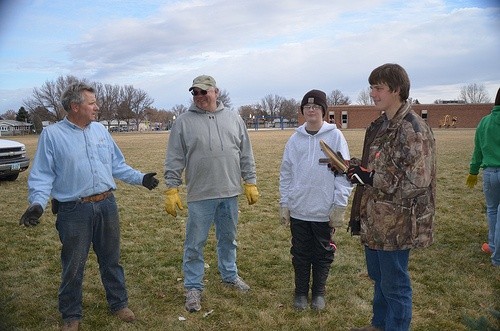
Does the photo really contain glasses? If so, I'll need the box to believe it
[191,90,215,96]
[302,105,322,110]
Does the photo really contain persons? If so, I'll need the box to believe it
[465,88,500,266]
[19,81,159,331]
[279,90,354,309]
[163,74,259,312]
[319,64,437,331]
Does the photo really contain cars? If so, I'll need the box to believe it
[110,127,128,132]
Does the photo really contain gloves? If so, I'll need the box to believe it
[465,174,478,189]
[280,207,291,226]
[244,183,260,205]
[164,187,183,217]
[329,206,348,227]
[328,152,354,174]
[142,173,159,190]
[19,203,43,226]
[346,166,375,187]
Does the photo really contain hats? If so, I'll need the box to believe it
[301,90,327,118]
[189,75,216,90]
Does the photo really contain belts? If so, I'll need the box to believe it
[75,191,111,203]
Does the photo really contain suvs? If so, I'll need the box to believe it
[0,139,30,181]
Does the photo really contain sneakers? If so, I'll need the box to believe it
[110,307,135,322]
[351,324,382,331]
[185,288,201,312]
[62,318,80,331]
[294,296,307,309]
[482,242,492,255]
[312,295,326,309]
[221,276,250,290]
